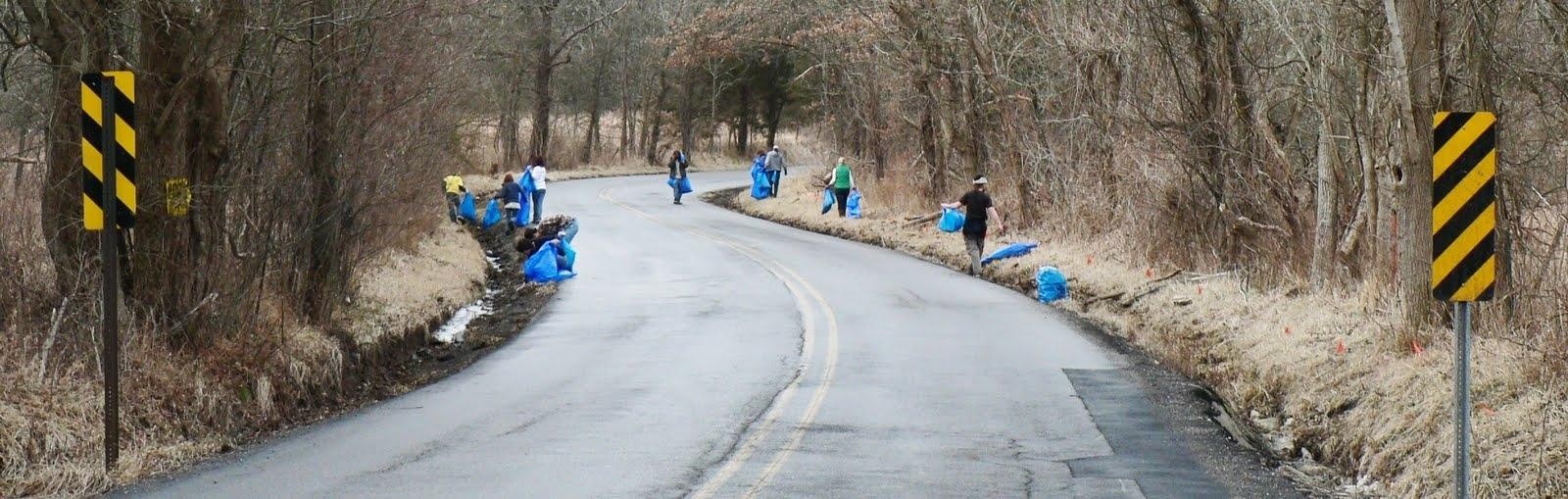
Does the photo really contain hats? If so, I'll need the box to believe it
[773,145,779,150]
[972,175,989,186]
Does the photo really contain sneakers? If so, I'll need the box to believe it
[451,215,543,236]
[671,200,683,205]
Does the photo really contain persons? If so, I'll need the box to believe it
[516,230,568,267]
[824,157,857,219]
[941,174,1004,279]
[442,172,467,226]
[494,175,529,235]
[525,214,577,248]
[526,156,547,224]
[764,144,787,197]
[756,147,766,157]
[667,151,688,204]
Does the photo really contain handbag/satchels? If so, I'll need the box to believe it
[823,172,834,186]
